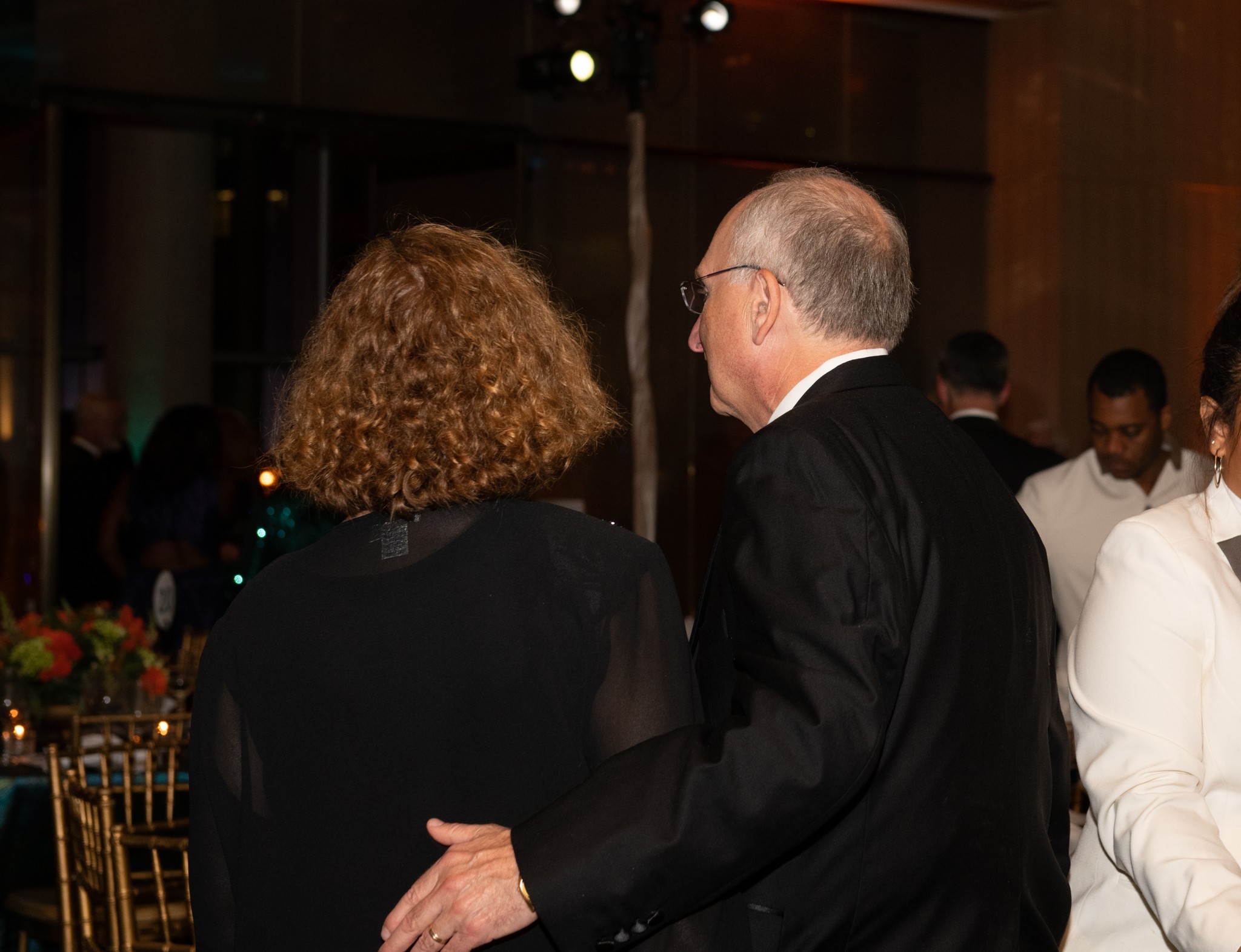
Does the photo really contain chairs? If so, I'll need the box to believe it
[0,713,196,951]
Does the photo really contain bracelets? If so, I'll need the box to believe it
[518,875,535,913]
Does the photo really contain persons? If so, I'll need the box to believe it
[189,218,704,952]
[377,167,1071,952]
[56,351,254,637]
[931,286,1240,952]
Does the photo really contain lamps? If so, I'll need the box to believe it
[516,0,734,98]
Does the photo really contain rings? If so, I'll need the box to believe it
[427,926,449,944]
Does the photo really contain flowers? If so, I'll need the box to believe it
[1,597,171,703]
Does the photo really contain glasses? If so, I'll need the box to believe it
[680,265,785,315]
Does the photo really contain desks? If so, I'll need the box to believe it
[0,770,193,952]
